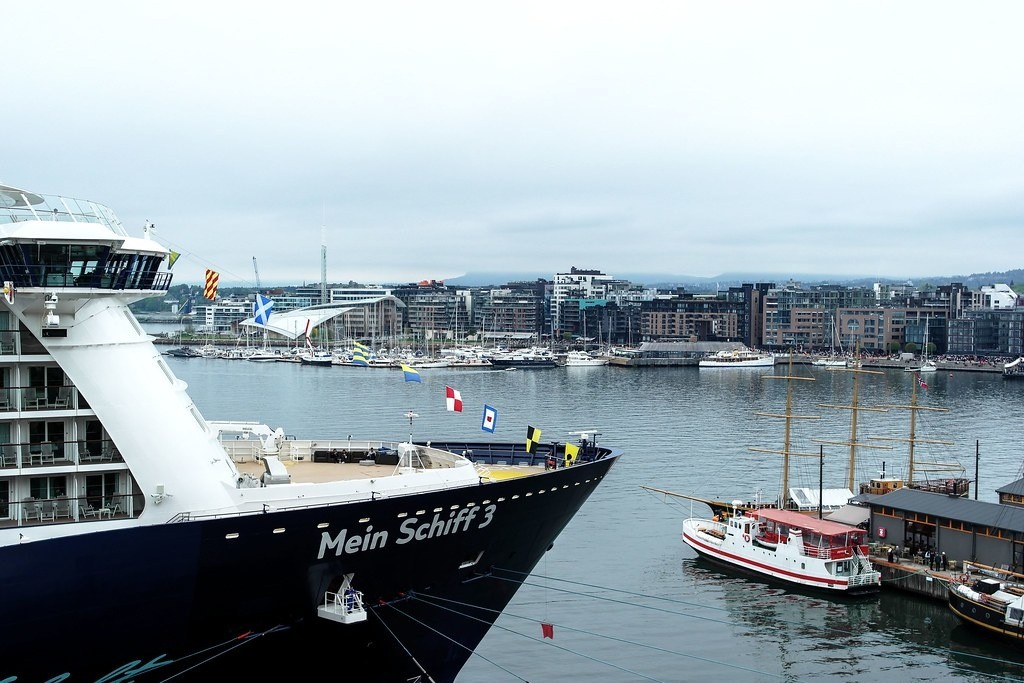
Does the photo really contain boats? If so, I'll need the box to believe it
[0,180,629,683]
[698,345,776,367]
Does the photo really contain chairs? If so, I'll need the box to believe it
[868,545,924,566]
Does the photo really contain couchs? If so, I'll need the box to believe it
[314,451,379,463]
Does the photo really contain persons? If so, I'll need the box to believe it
[886,546,948,572]
[562,454,573,467]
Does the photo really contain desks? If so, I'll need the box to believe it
[879,547,890,556]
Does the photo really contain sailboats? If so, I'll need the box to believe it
[637,334,1024,598]
[165,291,618,369]
[810,315,847,366]
[947,461,1024,642]
[846,325,863,369]
[920,314,937,372]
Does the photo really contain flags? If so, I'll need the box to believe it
[401,363,421,384]
[446,385,462,413]
[306,318,315,351]
[526,425,541,454]
[203,269,220,302]
[564,442,579,467]
[353,340,372,370]
[482,404,497,433]
[254,293,274,326]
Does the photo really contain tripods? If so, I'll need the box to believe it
[391,410,427,477]
